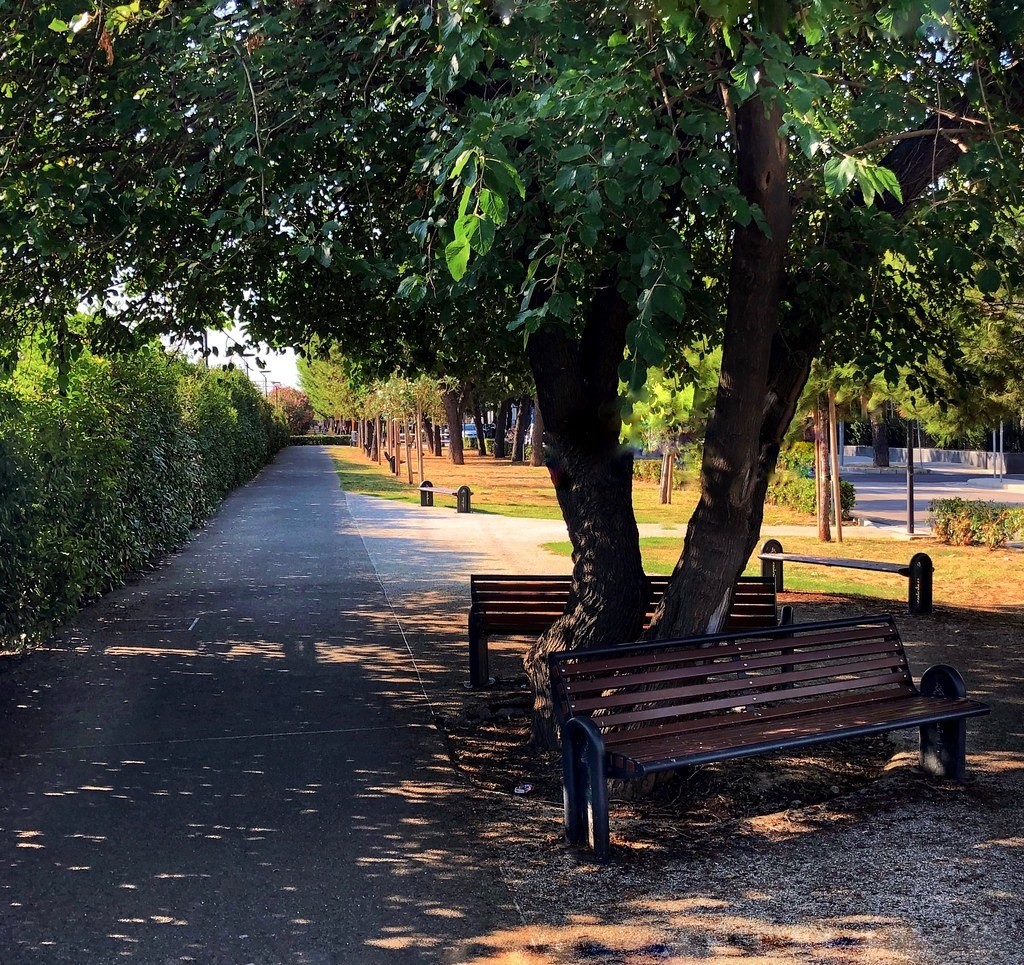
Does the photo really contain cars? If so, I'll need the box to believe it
[463,423,485,439]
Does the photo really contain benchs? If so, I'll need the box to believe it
[417,480,474,513]
[470,574,795,686]
[549,614,991,857]
[759,539,936,614]
